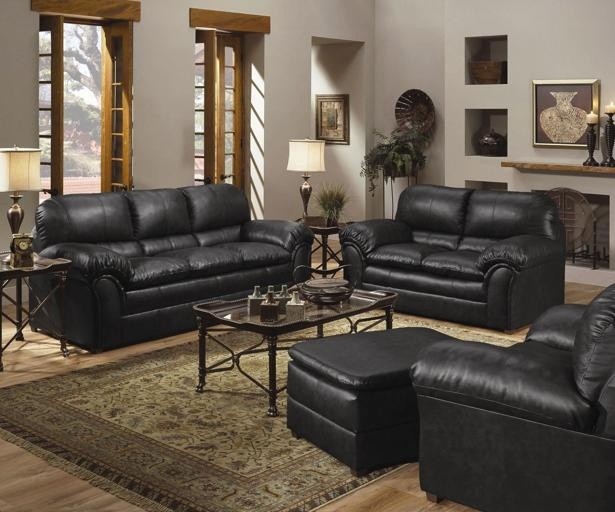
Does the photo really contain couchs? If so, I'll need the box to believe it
[29,183,314,353]
[340,185,565,332]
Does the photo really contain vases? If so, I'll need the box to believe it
[479,127,507,155]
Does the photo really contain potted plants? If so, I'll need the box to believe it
[314,186,346,227]
[359,122,425,221]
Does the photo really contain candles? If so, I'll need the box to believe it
[586,108,598,122]
[604,102,615,114]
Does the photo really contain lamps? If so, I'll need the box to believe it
[0,144,41,253]
[287,138,323,218]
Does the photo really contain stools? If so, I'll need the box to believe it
[286,325,455,473]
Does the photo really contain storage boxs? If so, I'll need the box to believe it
[469,60,505,83]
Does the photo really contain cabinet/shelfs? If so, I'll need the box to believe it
[464,35,507,192]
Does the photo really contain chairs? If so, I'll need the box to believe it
[411,284,614,511]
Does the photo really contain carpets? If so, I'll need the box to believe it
[0,298,524,512]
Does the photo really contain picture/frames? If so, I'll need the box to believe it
[532,79,600,148]
[316,94,350,145]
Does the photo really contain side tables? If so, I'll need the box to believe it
[0,250,72,371]
[300,217,346,279]
